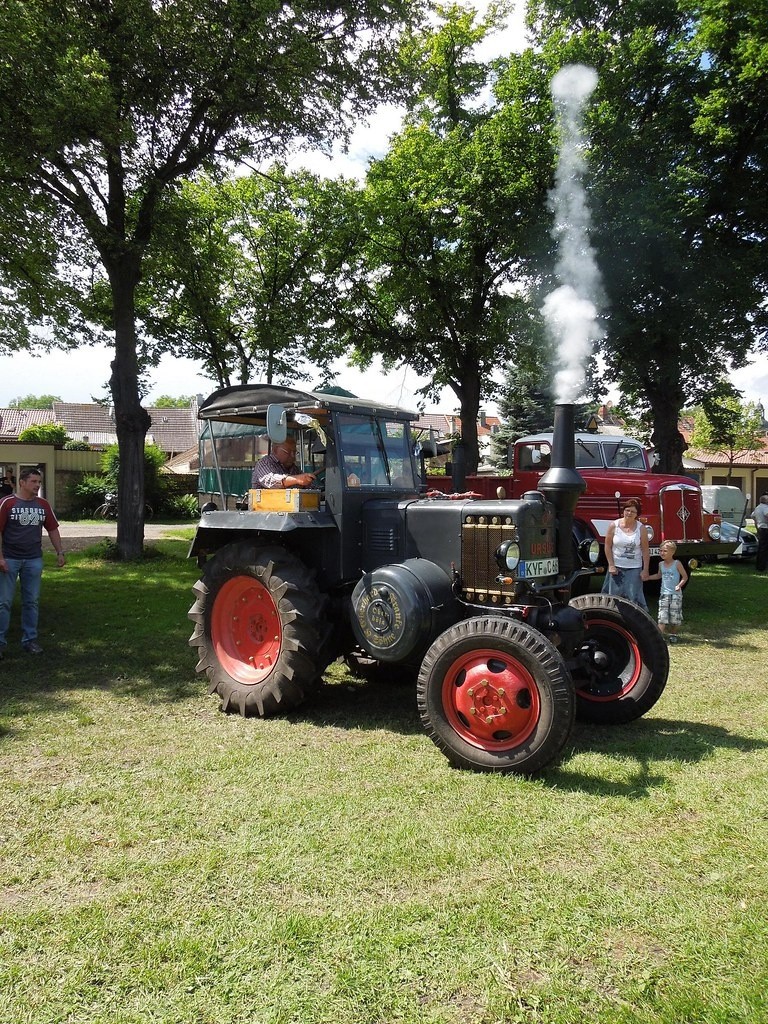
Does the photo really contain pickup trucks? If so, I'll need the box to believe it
[424,432,741,593]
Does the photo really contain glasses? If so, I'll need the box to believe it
[279,447,299,456]
[625,509,637,514]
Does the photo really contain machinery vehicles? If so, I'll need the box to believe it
[186,383,670,774]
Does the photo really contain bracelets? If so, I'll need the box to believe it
[57,552,64,556]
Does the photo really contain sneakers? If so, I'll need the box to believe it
[0,646,4,660]
[26,642,43,654]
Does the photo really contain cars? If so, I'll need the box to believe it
[720,521,758,560]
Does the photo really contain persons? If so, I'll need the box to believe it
[750,495,768,572]
[250,433,324,492]
[642,540,688,644]
[0,470,16,499]
[0,467,66,660]
[601,499,649,612]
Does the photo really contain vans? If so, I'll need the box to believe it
[701,485,747,528]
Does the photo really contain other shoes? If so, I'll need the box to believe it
[670,634,677,643]
